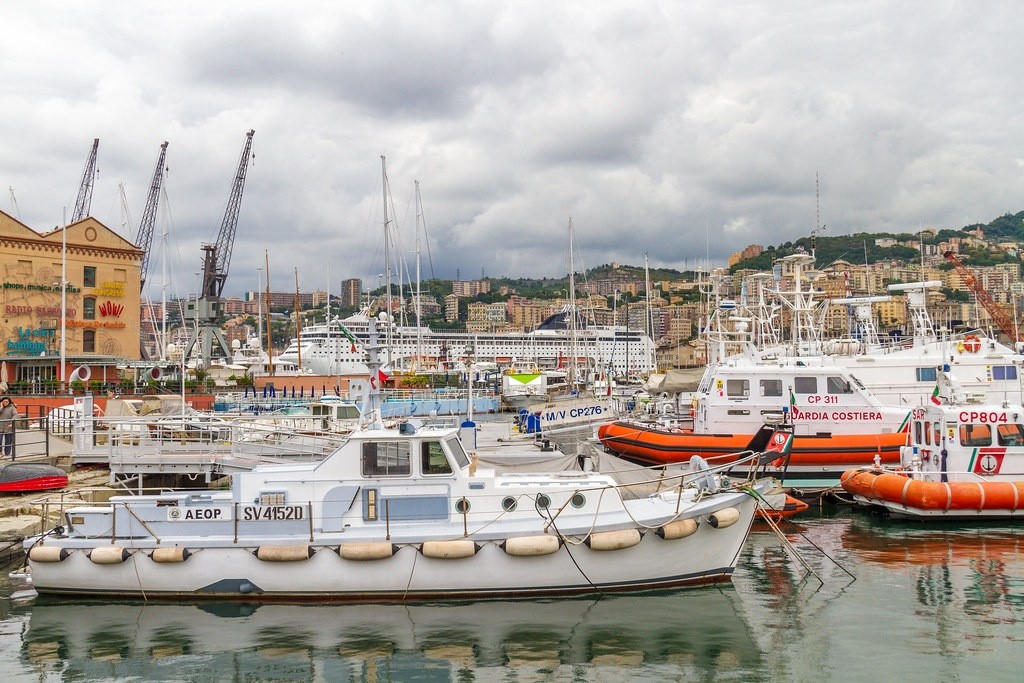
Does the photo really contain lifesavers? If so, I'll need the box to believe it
[963,335,982,353]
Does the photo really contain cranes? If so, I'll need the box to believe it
[134,141,169,360]
[69,138,100,225]
[183,128,255,366]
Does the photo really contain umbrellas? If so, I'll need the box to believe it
[0,361,9,392]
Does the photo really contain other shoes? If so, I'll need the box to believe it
[0,453,4,457]
[0,455,11,459]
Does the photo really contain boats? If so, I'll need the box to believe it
[20,584,797,683]
[276,297,656,377]
[596,221,1024,496]
[842,507,1024,654]
[840,350,1023,520]
[472,439,810,523]
[24,310,770,598]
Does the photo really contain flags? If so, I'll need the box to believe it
[606,372,612,396]
[931,375,942,405]
[376,362,393,384]
[791,391,799,420]
[336,320,358,353]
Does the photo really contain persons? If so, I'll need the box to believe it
[0,397,19,455]
